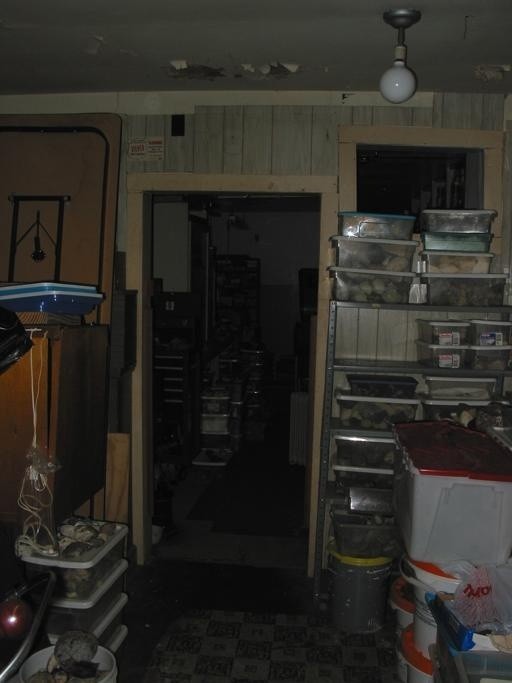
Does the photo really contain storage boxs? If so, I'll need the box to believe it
[328,375,512,569]
[328,208,512,375]
[21,519,129,666]
[200,350,267,449]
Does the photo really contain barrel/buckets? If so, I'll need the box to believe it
[387,577,414,636]
[20,644,119,683]
[398,553,463,661]
[325,539,393,635]
[398,622,434,682]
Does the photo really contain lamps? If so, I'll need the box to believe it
[379,7,422,104]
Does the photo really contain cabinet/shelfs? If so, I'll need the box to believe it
[0,325,106,527]
